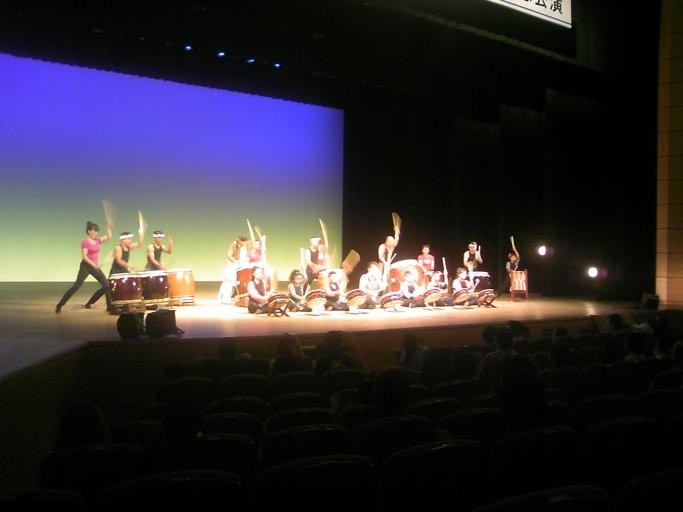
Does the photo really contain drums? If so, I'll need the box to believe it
[109,272,142,307]
[477,288,496,302]
[509,269,529,294]
[317,268,348,301]
[236,264,264,295]
[422,287,442,303]
[138,269,169,303]
[267,293,289,310]
[305,289,327,308]
[381,291,404,307]
[467,271,492,293]
[452,288,470,302]
[426,270,444,289]
[162,268,196,300]
[345,289,367,305]
[387,259,426,299]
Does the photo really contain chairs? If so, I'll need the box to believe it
[0,322,680,512]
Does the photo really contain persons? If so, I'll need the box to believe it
[357,260,384,308]
[450,267,481,305]
[463,242,483,271]
[504,246,521,297]
[56,221,174,314]
[304,236,331,295]
[324,272,349,312]
[220,235,263,303]
[285,269,311,313]
[246,266,275,312]
[399,268,452,307]
[378,228,400,269]
[417,244,435,274]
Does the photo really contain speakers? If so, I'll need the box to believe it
[146,309,175,336]
[116,311,144,338]
[640,293,658,308]
